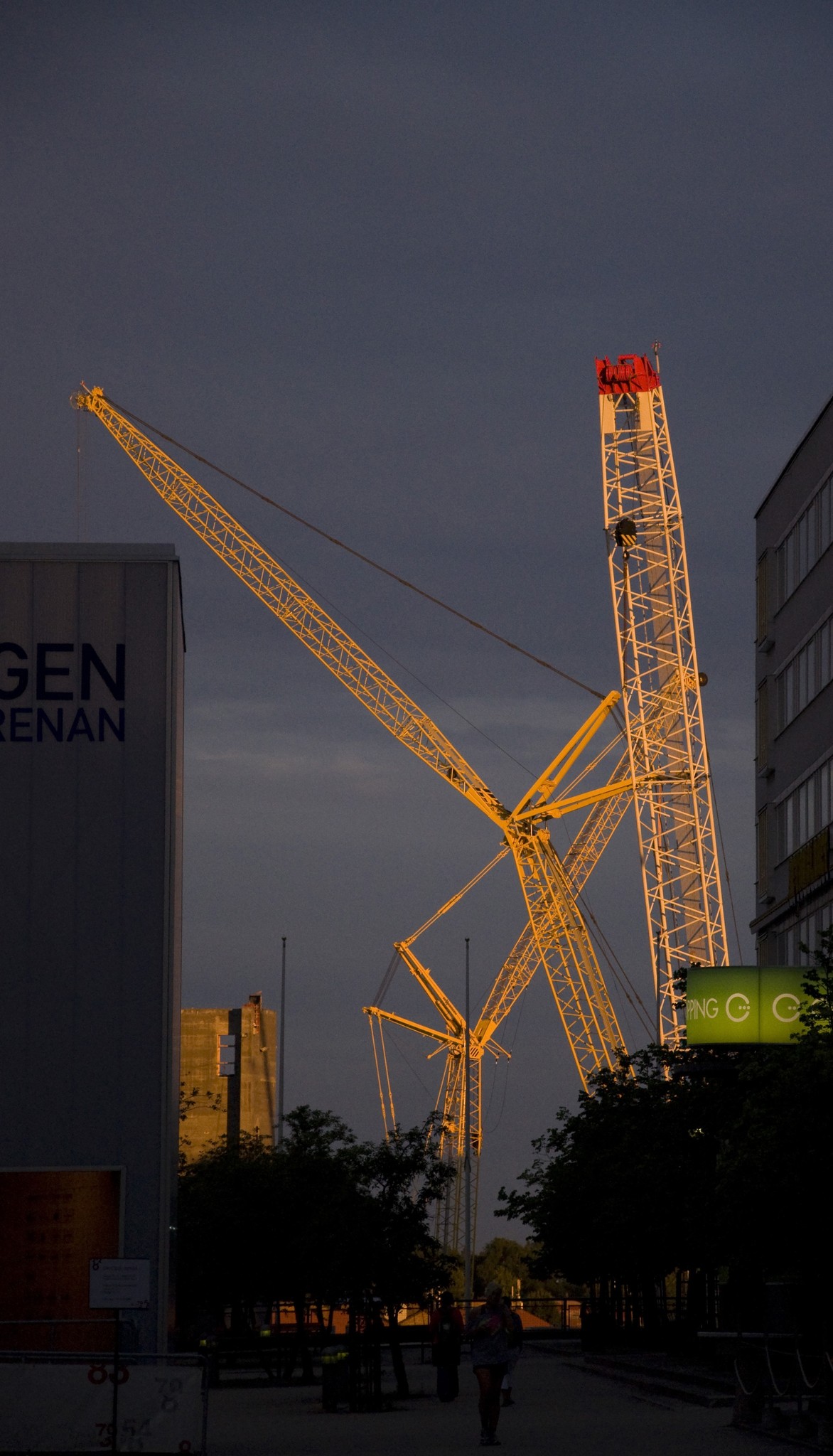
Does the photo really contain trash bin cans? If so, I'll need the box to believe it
[321,1332,383,1413]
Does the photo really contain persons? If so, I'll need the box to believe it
[428,1290,464,1403]
[498,1296,524,1408]
[464,1282,519,1446]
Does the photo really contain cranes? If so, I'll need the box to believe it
[69,337,727,1301]
